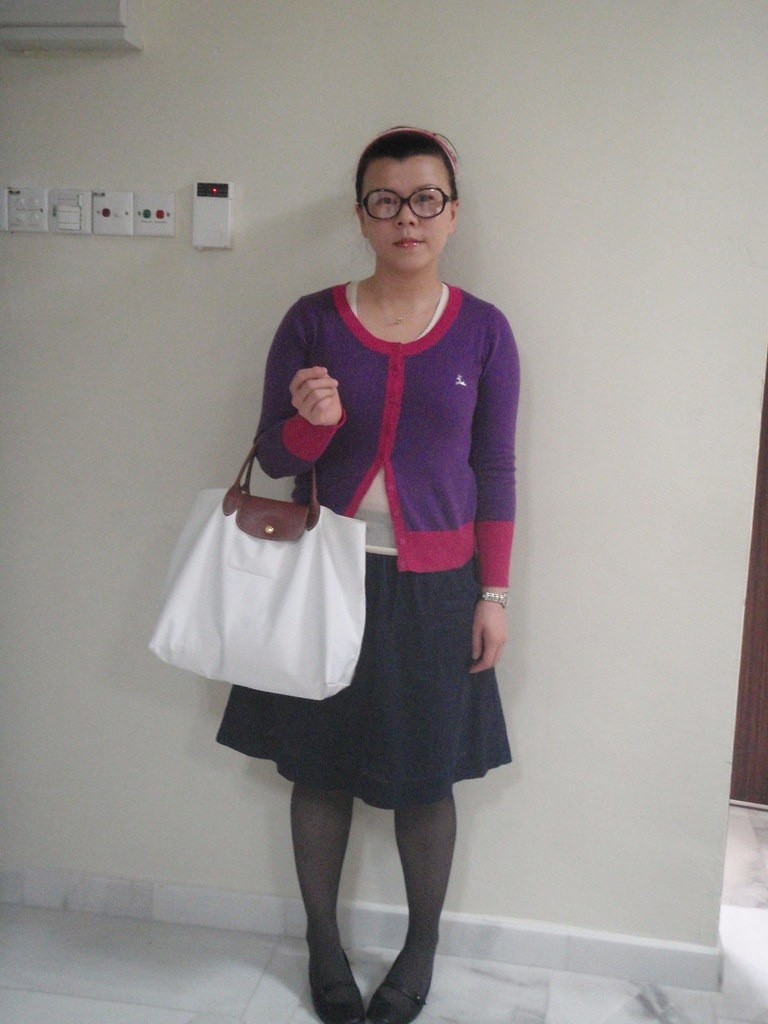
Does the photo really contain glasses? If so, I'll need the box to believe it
[355,189,457,219]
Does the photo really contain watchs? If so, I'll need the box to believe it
[480,592,509,608]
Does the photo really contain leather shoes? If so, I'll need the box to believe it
[366,948,433,1024]
[308,948,365,1024]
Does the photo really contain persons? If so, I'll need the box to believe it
[217,120,521,1024]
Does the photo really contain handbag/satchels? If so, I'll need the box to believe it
[149,420,367,701]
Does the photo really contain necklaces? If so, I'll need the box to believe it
[389,305,418,325]
